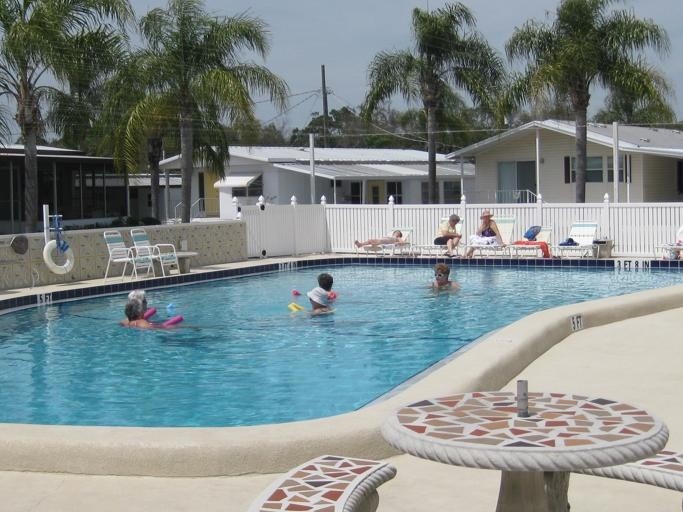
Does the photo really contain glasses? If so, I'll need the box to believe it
[434,273,446,278]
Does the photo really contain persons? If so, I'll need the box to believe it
[121,299,184,330]
[129,289,173,313]
[292,273,339,300]
[355,230,404,250]
[429,263,461,291]
[460,211,503,258]
[288,288,337,319]
[433,215,462,257]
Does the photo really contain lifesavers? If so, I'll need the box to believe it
[42,240,74,275]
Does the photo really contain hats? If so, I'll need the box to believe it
[479,210,493,220]
[305,286,332,308]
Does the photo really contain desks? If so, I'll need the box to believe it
[175,251,197,273]
[376,389,670,511]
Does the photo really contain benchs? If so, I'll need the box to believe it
[569,447,682,511]
[249,454,397,512]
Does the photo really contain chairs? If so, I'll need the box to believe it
[102,228,155,282]
[654,240,682,260]
[129,226,181,278]
[355,217,599,259]
